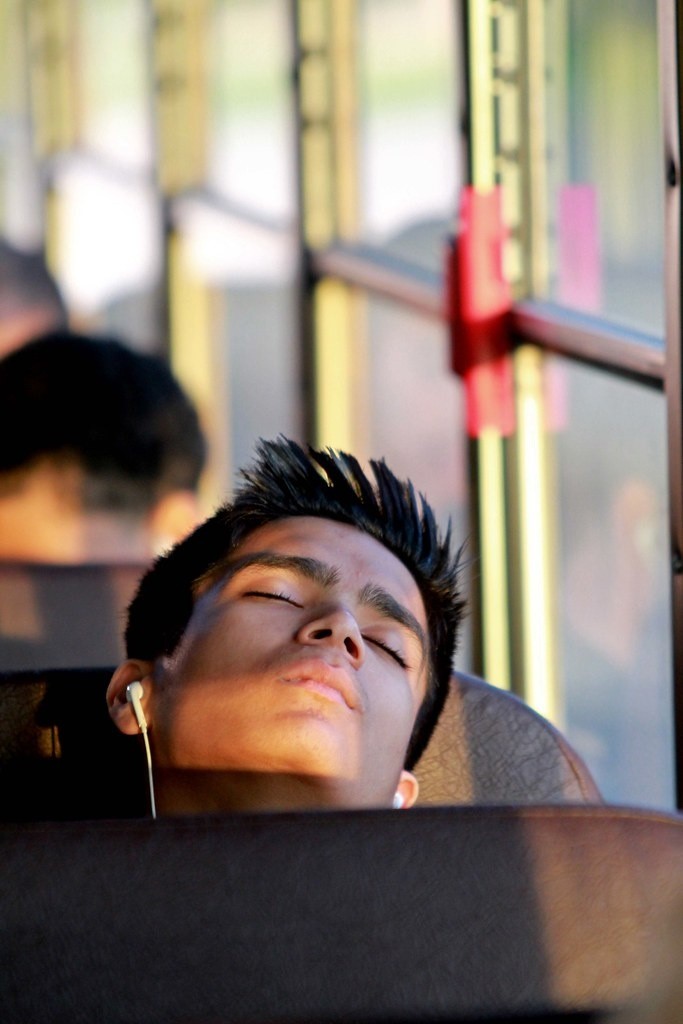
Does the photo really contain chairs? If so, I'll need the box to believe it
[0,807,681,1023]
[2,671,606,821]
[0,559,153,673]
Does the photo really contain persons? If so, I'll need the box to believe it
[102,433,469,821]
[1,241,64,356]
[1,331,206,567]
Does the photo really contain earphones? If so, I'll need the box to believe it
[392,793,403,809]
[126,681,147,727]
